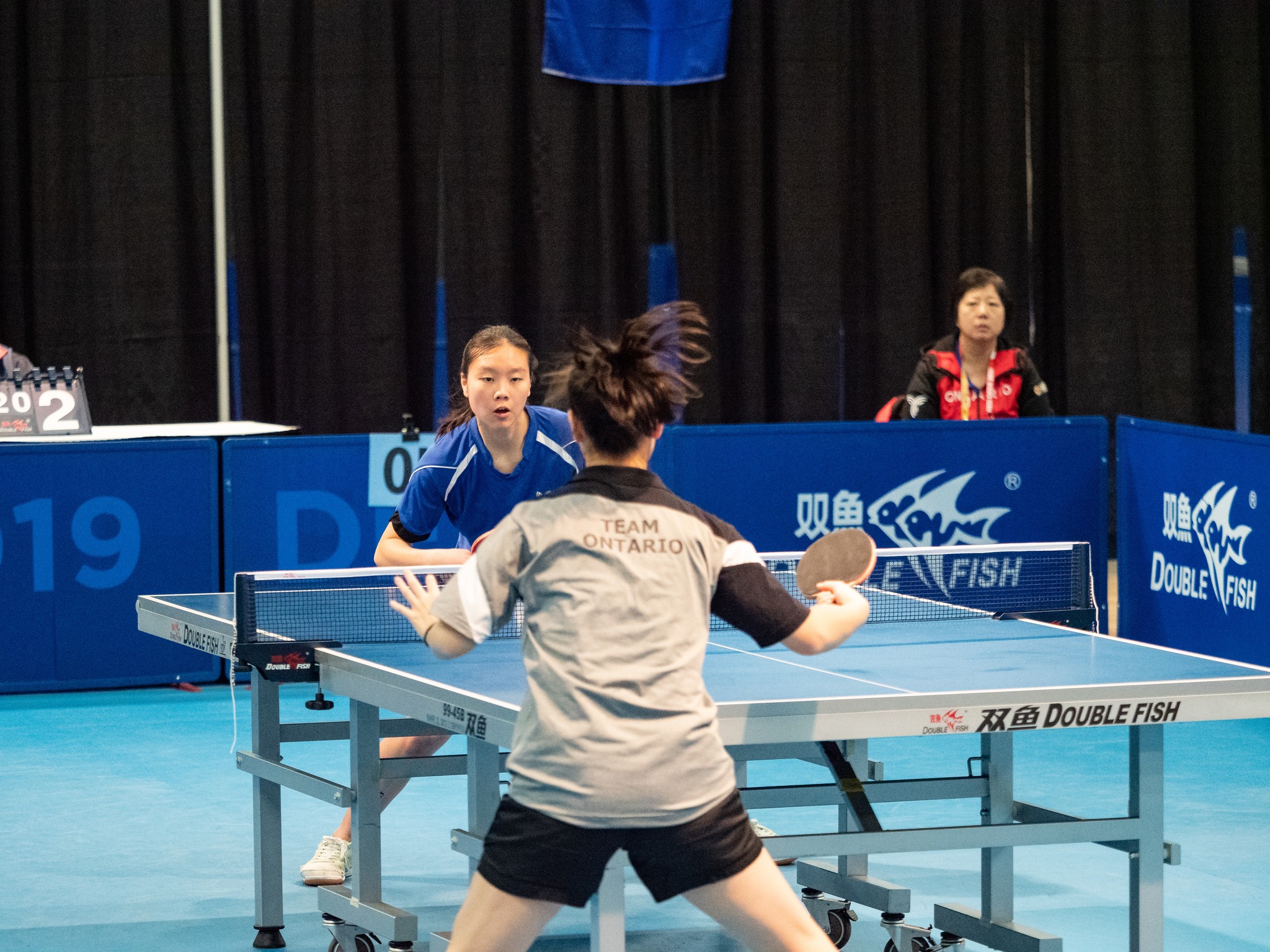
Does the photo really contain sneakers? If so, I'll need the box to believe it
[300,835,354,884]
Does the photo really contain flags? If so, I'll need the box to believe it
[541,0,732,86]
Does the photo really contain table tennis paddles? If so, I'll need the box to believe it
[797,529,877,605]
[471,529,493,555]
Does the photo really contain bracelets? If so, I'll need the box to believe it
[424,622,436,647]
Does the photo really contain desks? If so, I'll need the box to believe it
[137,569,1270,952]
[0,420,300,441]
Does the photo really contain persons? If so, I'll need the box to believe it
[902,267,1055,420]
[298,325,585,883]
[0,344,34,382]
[388,300,870,951]
